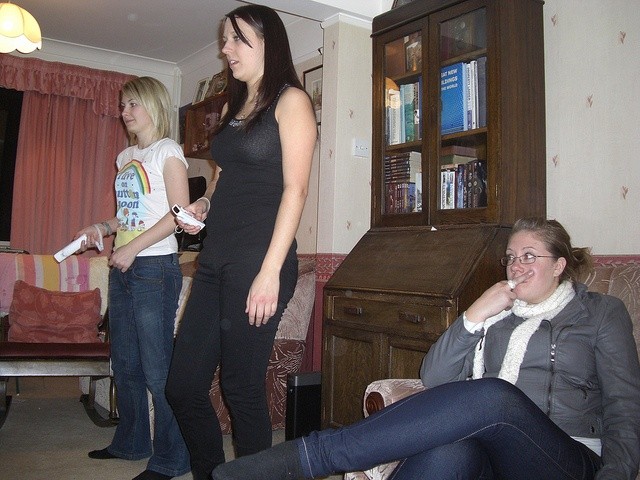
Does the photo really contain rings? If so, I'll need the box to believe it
[507,279,516,291]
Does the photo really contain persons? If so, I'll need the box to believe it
[70,76,192,479]
[211,218,640,480]
[164,4,319,479]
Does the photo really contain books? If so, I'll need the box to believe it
[0,245,31,255]
[380,57,488,148]
[381,7,494,79]
[375,150,489,217]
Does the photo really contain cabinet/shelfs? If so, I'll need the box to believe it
[370,1,547,232]
[321,227,515,428]
[184,90,229,160]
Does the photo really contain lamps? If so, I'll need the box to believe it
[0,0,43,56]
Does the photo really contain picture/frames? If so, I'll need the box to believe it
[191,77,208,105]
[302,63,322,126]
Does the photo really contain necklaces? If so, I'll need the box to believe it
[236,97,258,121]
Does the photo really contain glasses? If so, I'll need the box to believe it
[500,255,559,267]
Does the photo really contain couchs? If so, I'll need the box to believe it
[1,253,317,432]
[343,251,640,479]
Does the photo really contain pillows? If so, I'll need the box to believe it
[7,280,102,344]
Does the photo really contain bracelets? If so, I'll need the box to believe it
[100,221,113,236]
[197,196,211,214]
